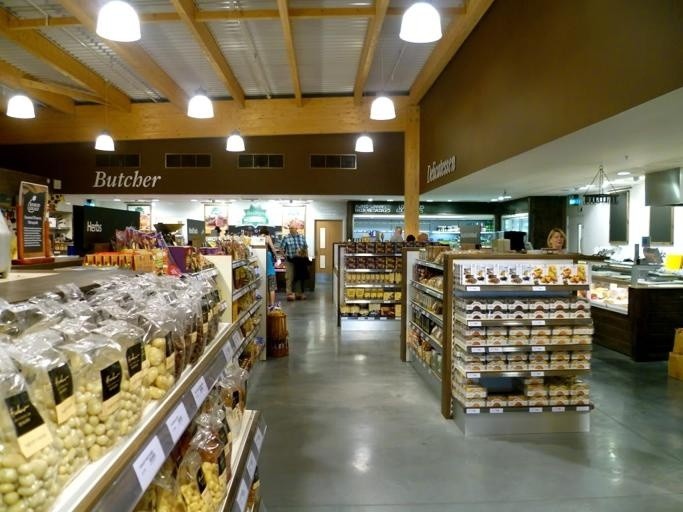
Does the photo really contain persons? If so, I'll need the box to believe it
[279,224,308,301]
[390,227,403,242]
[255,229,277,306]
[546,227,567,251]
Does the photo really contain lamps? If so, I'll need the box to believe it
[355,1,446,154]
[95,1,144,43]
[93,59,116,152]
[223,75,247,155]
[183,34,213,122]
[6,46,35,119]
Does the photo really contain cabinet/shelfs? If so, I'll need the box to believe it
[590,258,683,363]
[0,232,285,510]
[400,247,597,418]
[331,240,451,330]
